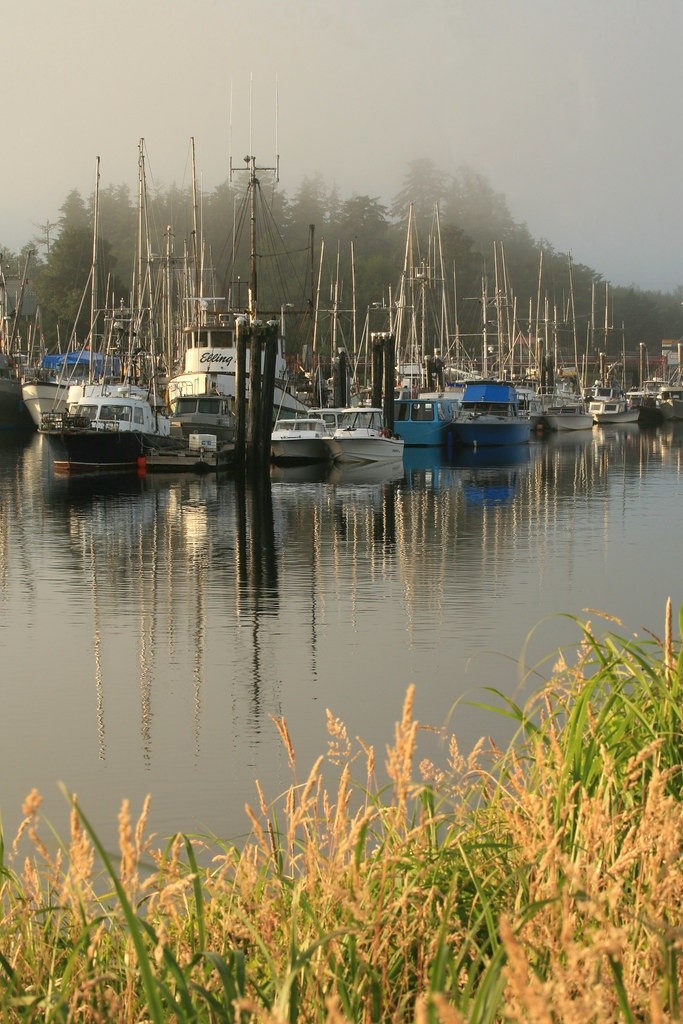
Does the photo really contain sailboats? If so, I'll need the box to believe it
[0,65,683,479]
